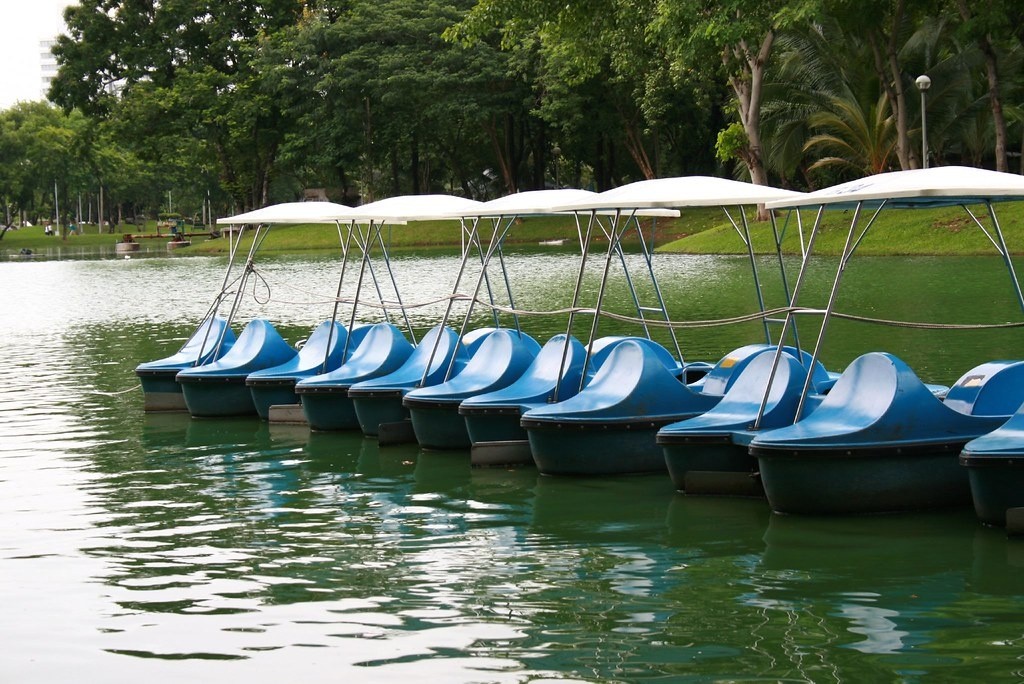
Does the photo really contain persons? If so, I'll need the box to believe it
[68,220,77,236]
[44,221,53,236]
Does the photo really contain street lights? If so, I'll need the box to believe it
[915,75,930,170]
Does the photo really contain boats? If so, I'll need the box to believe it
[132,166,1023,538]
